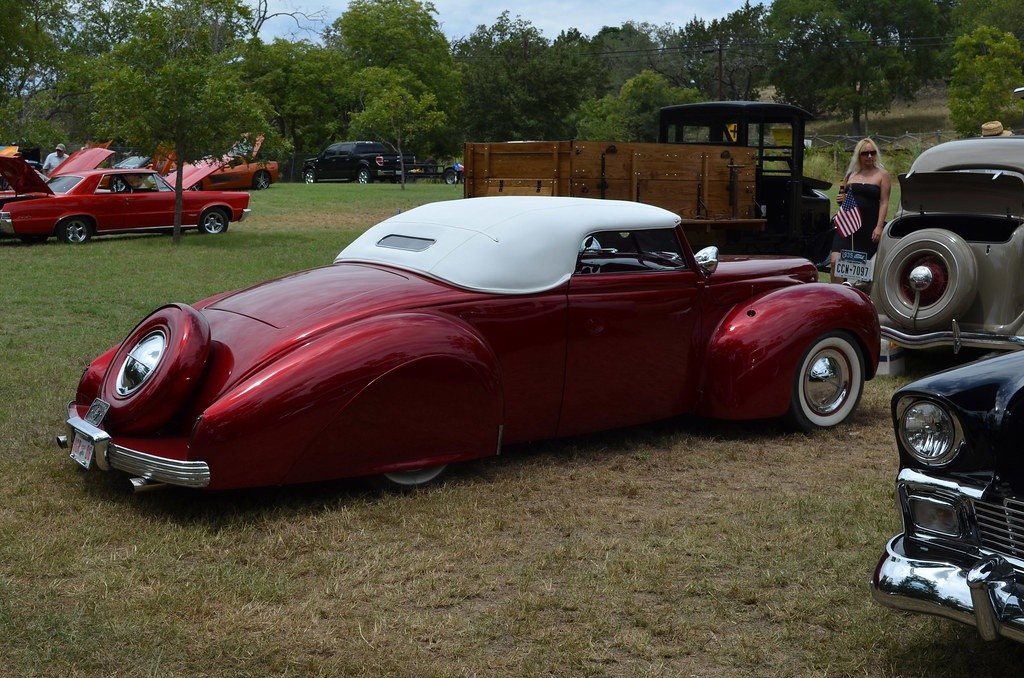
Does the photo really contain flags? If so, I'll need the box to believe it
[833,188,862,238]
[455,163,465,172]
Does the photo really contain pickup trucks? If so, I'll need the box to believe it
[300,141,417,185]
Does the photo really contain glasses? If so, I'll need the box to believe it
[860,150,876,157]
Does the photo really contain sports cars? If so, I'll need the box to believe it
[54,194,883,505]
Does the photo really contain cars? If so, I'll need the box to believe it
[867,134,1023,365]
[868,348,1023,655]
[0,129,278,247]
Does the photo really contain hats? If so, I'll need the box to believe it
[980,121,1012,137]
[55,143,65,152]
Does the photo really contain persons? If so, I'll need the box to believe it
[829,138,892,284]
[43,144,70,177]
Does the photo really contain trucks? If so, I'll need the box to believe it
[461,100,833,261]
